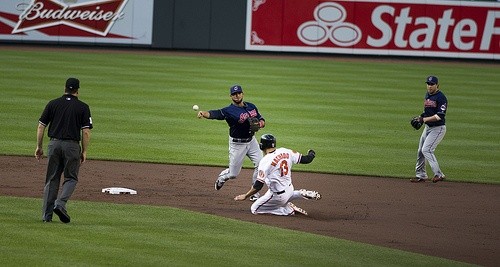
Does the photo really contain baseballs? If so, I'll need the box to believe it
[192,104,199,112]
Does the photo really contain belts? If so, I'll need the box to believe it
[233,137,252,143]
[277,184,291,195]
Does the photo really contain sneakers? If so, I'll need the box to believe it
[302,190,320,199]
[215,178,225,190]
[410,177,428,182]
[289,202,308,216]
[249,196,258,201]
[433,175,445,182]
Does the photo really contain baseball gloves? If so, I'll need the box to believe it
[248,117,260,135]
[410,116,423,130]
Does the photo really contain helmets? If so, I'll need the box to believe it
[259,134,276,150]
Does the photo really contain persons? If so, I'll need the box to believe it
[234,134,322,216]
[35,77,93,223]
[197,85,266,202]
[410,75,448,183]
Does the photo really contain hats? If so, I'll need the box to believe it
[230,86,243,95]
[66,78,80,89]
[425,76,438,85]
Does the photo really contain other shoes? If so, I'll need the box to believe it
[53,205,71,223]
[43,220,52,223]
